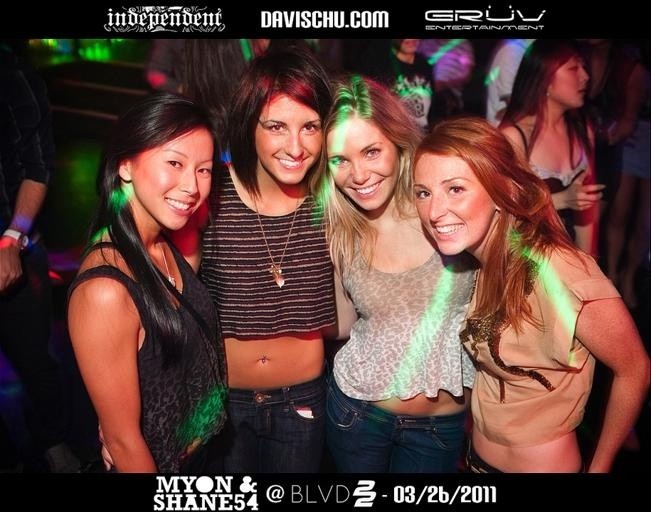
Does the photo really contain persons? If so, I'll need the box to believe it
[1,37,651,474]
